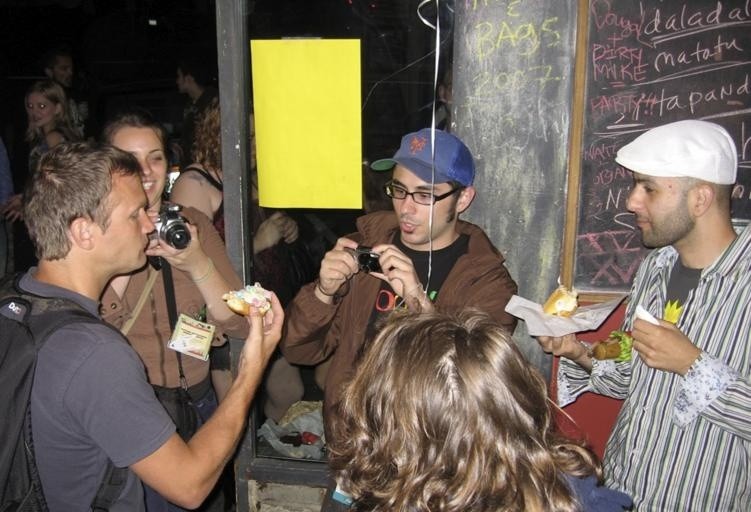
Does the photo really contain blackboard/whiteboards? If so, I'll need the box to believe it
[448,2,578,391]
[569,0,751,304]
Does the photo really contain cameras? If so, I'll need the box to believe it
[147,203,192,251]
[344,245,395,274]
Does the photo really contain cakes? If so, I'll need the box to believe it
[223,283,270,317]
[542,286,577,317]
[279,400,325,443]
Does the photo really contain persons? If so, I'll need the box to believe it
[3,52,97,275]
[102,109,247,512]
[168,60,392,422]
[1,138,290,511]
[528,119,751,511]
[320,300,639,512]
[284,127,520,512]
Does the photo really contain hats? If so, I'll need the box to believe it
[614,120,738,185]
[372,127,476,188]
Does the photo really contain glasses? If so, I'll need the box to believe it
[384,179,459,206]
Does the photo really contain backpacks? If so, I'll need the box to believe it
[2,274,136,510]
